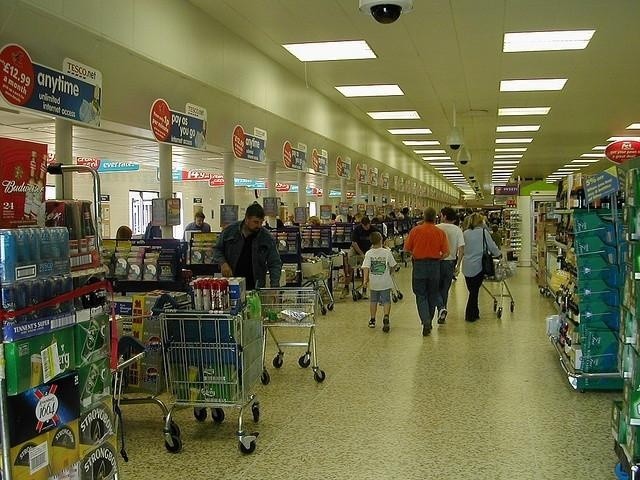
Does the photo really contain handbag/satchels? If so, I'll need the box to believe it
[481,252,495,278]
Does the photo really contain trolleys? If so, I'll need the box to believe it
[481,253,517,318]
[300,254,335,315]
[261,284,326,386]
[160,288,265,456]
[348,247,405,304]
[106,308,183,460]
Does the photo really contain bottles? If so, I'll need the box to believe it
[505,199,519,220]
[554,178,625,210]
[92,85,101,126]
[202,121,206,140]
[0,225,70,285]
[23,150,38,220]
[141,319,165,393]
[90,365,106,403]
[31,152,47,219]
[63,201,96,254]
[554,224,581,372]
[94,320,107,354]
[193,278,231,313]
[262,140,267,154]
[246,287,263,322]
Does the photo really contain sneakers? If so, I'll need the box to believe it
[339,286,447,336]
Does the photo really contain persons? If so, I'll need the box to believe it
[213,203,282,290]
[283,213,298,225]
[332,207,502,336]
[183,212,210,240]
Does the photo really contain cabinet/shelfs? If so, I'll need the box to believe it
[102,213,445,395]
[0,162,117,480]
[506,154,640,479]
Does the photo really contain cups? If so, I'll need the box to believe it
[194,134,205,148]
[259,151,266,161]
[30,355,44,387]
[79,99,96,122]
[188,366,199,381]
[191,388,200,401]
